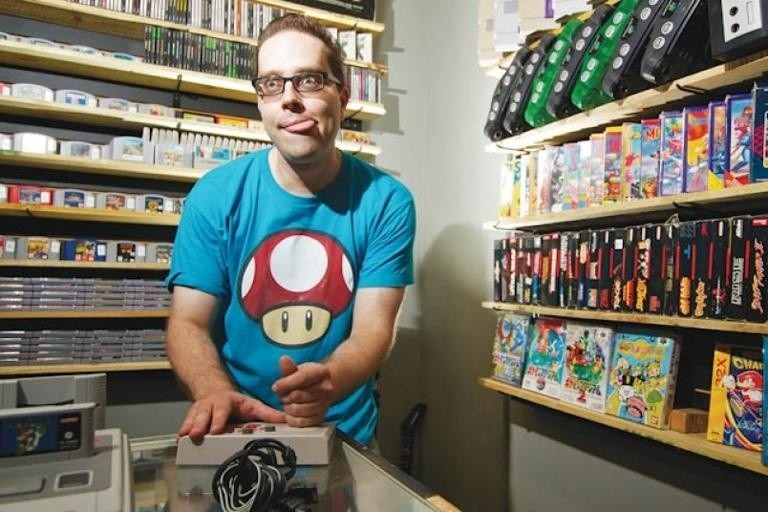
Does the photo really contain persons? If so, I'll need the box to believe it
[164,12,415,455]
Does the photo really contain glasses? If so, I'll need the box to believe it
[251,71,343,97]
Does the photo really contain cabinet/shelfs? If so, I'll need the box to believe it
[480,38,737,459]
[0,0,390,395]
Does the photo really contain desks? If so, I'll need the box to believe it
[98,423,466,512]
